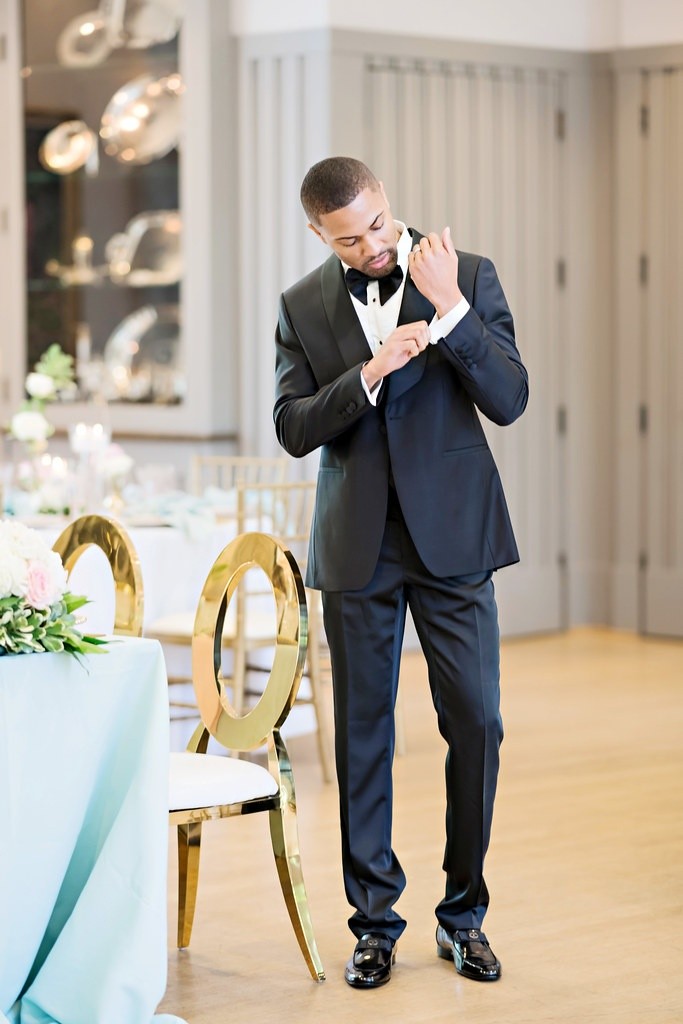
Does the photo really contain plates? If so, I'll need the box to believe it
[38,119,94,176]
[99,73,180,167]
[54,9,110,69]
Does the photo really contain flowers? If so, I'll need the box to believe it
[0,342,108,656]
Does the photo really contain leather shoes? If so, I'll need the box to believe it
[345,931,396,988]
[435,919,501,980]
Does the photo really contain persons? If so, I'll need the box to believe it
[273,157,531,990]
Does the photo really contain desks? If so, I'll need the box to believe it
[0,631,188,1024]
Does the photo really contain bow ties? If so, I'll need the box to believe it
[346,264,404,305]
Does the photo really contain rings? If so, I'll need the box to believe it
[413,249,421,257]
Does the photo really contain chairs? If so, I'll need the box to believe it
[165,530,327,987]
[112,444,336,787]
[46,513,146,640]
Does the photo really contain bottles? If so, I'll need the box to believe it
[59,237,105,291]
